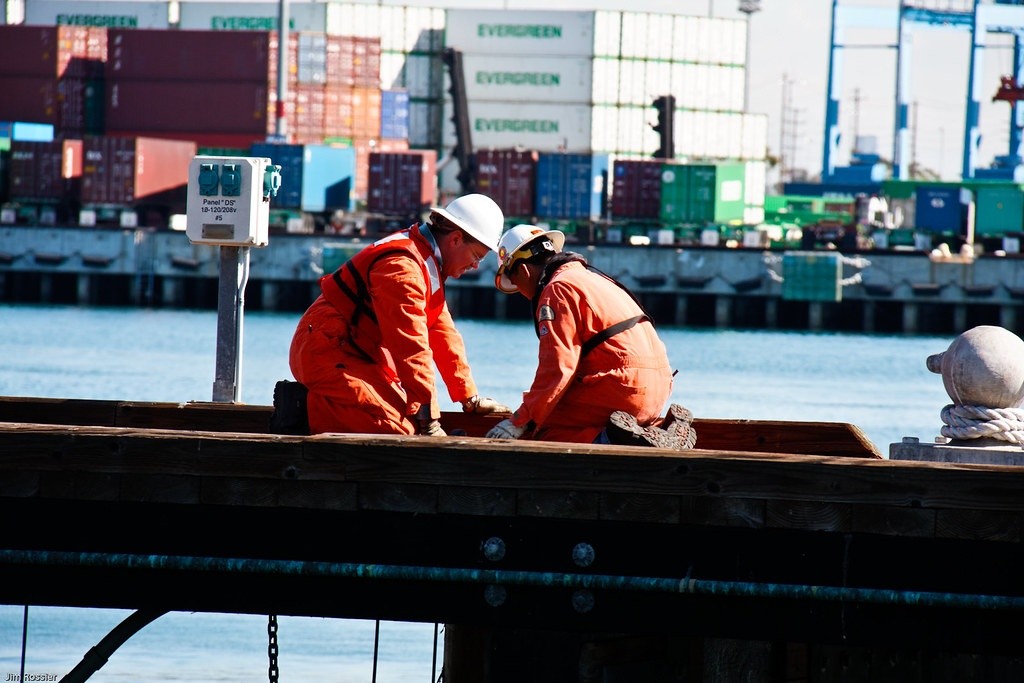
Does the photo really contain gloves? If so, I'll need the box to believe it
[464,396,512,413]
[423,420,447,436]
[486,418,525,439]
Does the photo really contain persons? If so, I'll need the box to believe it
[484,224,697,450]
[273,193,514,439]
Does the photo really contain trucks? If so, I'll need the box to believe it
[799,197,859,254]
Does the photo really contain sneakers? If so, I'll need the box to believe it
[268,379,305,434]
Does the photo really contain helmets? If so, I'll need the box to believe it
[495,224,565,294]
[430,193,504,253]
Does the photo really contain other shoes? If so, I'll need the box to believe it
[662,404,697,450]
[607,411,683,450]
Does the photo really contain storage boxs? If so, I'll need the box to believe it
[0,0,1024,257]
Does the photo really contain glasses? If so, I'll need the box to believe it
[465,239,485,263]
